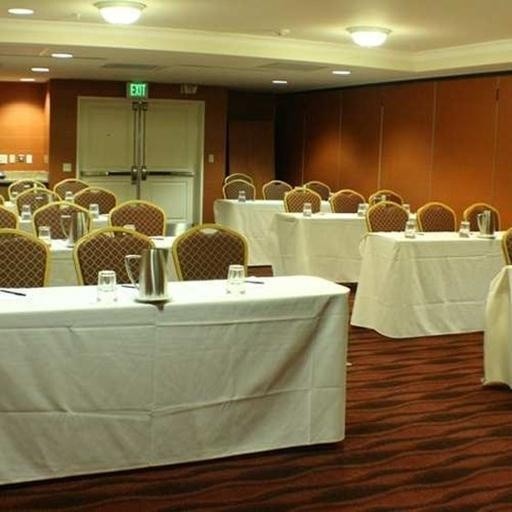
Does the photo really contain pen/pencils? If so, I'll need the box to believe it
[244,280,263,284]
[121,285,135,288]
[0,289,26,296]
[150,238,164,240]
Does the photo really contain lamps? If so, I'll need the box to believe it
[347,25,391,48]
[94,1,147,25]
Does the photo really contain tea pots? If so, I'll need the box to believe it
[477,207,496,236]
[124,246,171,304]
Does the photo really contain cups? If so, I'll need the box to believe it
[403,221,419,238]
[96,270,118,302]
[458,221,471,237]
[225,264,248,296]
[237,187,410,215]
[2,182,188,244]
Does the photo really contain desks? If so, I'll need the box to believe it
[363,229,512,341]
[0,276,349,487]
